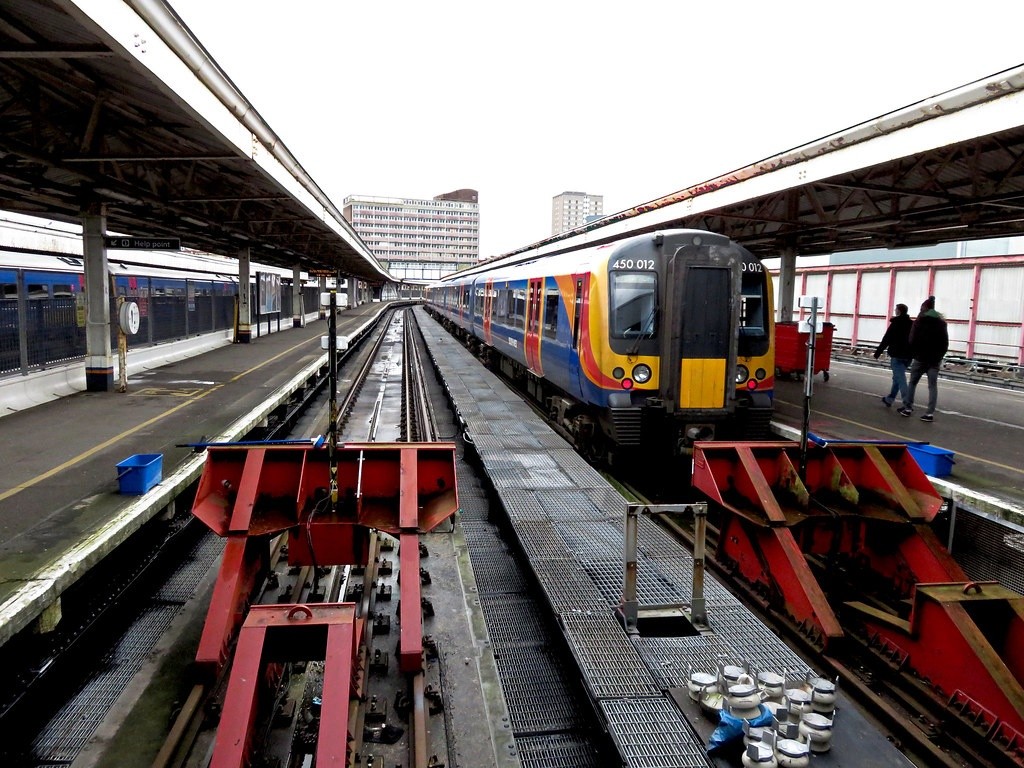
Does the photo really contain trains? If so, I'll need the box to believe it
[423,228,779,474]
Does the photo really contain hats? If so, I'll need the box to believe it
[922,296,935,309]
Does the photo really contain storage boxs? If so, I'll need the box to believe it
[906,443,956,478]
[114,453,164,497]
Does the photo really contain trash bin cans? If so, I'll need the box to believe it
[775,319,837,383]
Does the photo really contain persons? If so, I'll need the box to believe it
[897,296,948,422]
[874,304,915,417]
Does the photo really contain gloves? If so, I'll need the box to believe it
[873,346,884,359]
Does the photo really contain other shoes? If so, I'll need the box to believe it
[897,407,914,412]
[920,414,934,421]
[882,396,892,408]
[900,409,912,417]
